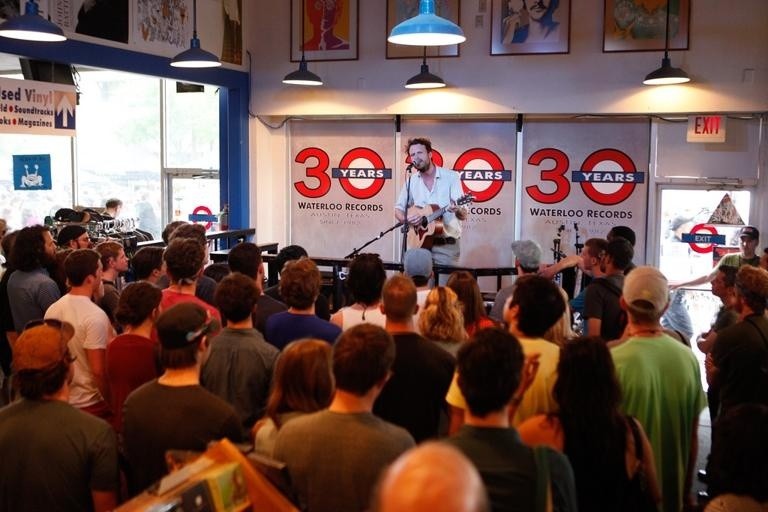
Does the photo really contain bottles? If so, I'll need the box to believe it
[738,227,759,238]
[404,248,433,278]
[12,322,74,373]
[58,225,86,245]
[157,301,221,346]
[511,240,541,269]
[623,266,669,314]
[669,215,695,230]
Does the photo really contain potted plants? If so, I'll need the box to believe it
[288,1,359,62]
[384,1,462,59]
[601,0,692,54]
[488,0,572,54]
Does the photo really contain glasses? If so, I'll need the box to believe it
[386,0,465,48]
[404,45,447,88]
[280,0,323,87]
[18,58,81,108]
[640,1,690,85]
[0,0,68,44]
[169,0,221,67]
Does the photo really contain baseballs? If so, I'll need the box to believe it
[217,203,228,231]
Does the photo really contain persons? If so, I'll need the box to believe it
[1,203,707,512]
[666,226,767,512]
[375,440,487,512]
[122,303,249,511]
[446,327,577,512]
[1,318,118,511]
[394,139,467,287]
[502,0,561,45]
[272,324,417,511]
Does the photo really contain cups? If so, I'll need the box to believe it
[24,319,63,329]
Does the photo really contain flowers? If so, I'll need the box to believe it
[407,159,423,170]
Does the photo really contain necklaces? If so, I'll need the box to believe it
[631,328,662,336]
